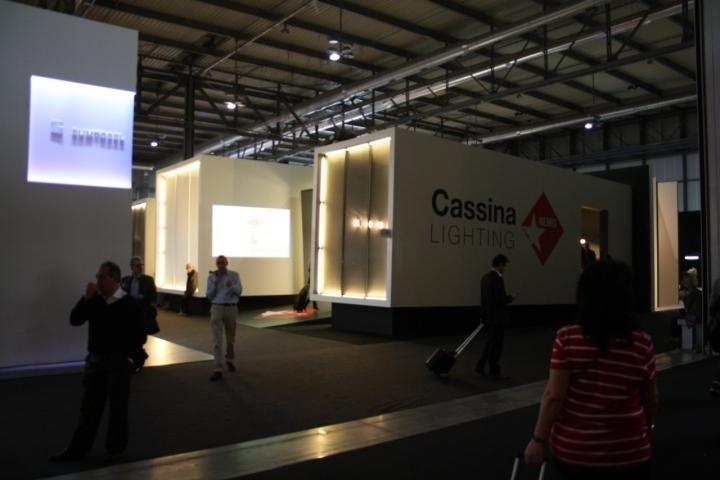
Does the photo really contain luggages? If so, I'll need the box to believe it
[425,323,485,378]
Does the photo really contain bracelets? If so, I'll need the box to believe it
[531,433,545,443]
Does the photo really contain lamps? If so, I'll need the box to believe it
[224,37,246,110]
[584,59,603,129]
[150,82,158,147]
[327,8,354,62]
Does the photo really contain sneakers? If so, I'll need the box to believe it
[225,361,236,372]
[47,448,88,461]
[210,372,223,382]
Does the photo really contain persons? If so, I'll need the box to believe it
[523,263,658,480]
[472,254,517,380]
[582,235,596,269]
[119,257,157,375]
[175,264,199,318]
[206,256,242,382]
[47,261,140,462]
